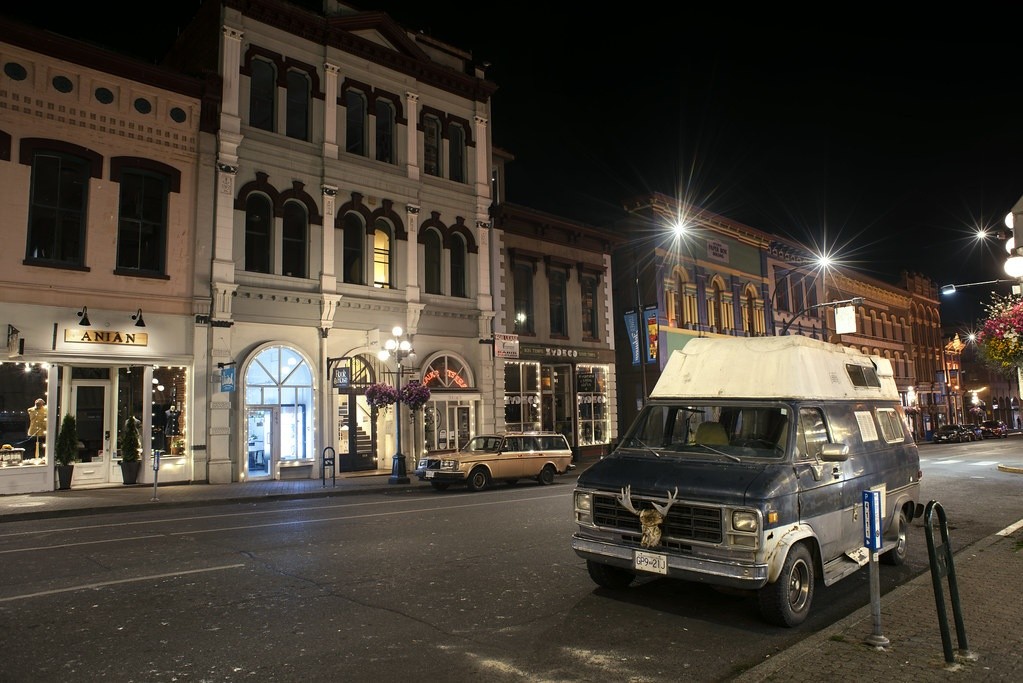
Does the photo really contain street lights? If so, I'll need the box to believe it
[771,257,833,335]
[633,225,683,409]
[906,385,918,441]
[378,326,419,483]
[971,391,980,424]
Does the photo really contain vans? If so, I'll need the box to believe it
[571,337,923,627]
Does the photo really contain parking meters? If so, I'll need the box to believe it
[864,491,889,645]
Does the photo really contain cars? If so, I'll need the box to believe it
[933,424,968,443]
[957,424,984,440]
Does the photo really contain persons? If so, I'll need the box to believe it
[164,405,181,437]
[512,442,520,451]
[585,417,602,442]
[1016,416,1022,429]
[27,399,47,436]
[125,415,141,436]
[494,441,500,450]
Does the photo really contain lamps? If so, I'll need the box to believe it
[710,289,724,299]
[77,307,90,326]
[132,309,146,327]
[744,297,757,306]
[670,281,686,291]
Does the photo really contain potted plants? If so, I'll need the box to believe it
[55,414,77,489]
[117,417,144,484]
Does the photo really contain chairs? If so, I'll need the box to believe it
[695,422,729,446]
[777,417,822,459]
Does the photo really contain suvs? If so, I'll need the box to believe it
[980,420,1008,439]
[413,430,576,491]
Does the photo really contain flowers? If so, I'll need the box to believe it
[401,381,431,409]
[364,382,400,408]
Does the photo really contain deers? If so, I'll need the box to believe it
[616,484,678,548]
